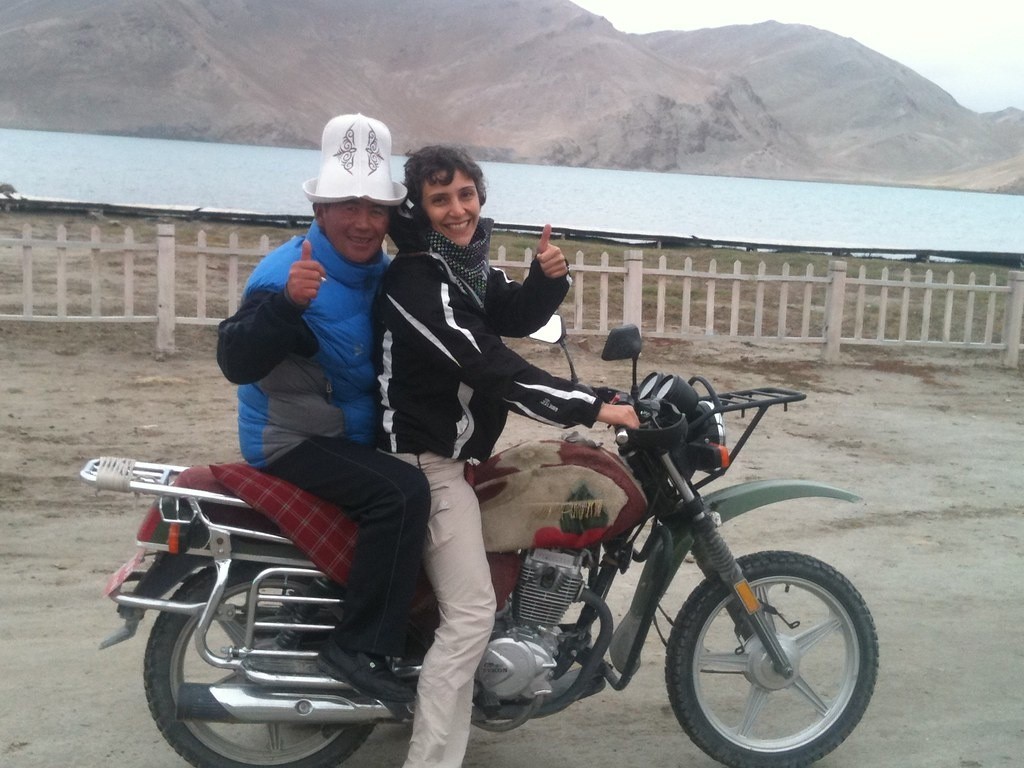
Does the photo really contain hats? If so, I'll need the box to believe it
[303,114,408,206]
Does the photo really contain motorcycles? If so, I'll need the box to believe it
[80,315,879,768]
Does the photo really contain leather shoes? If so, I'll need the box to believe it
[317,635,415,703]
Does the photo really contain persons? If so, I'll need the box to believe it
[216,112,431,704]
[373,144,641,767]
